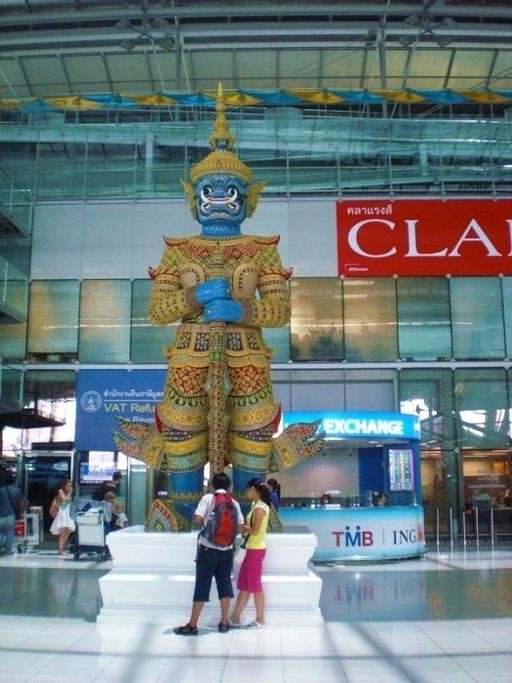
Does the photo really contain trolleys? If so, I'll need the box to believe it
[74,511,107,558]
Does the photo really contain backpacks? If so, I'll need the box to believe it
[198,492,240,546]
[48,495,61,518]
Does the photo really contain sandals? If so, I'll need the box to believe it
[172,623,199,635]
[217,622,232,632]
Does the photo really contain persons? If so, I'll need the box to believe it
[374,491,391,505]
[50,479,76,555]
[267,478,281,512]
[227,476,271,629]
[92,472,121,501]
[173,472,245,634]
[102,491,125,558]
[320,492,332,504]
[1,475,25,555]
[114,79,327,533]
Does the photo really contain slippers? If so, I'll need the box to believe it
[227,617,241,628]
[245,619,268,630]
